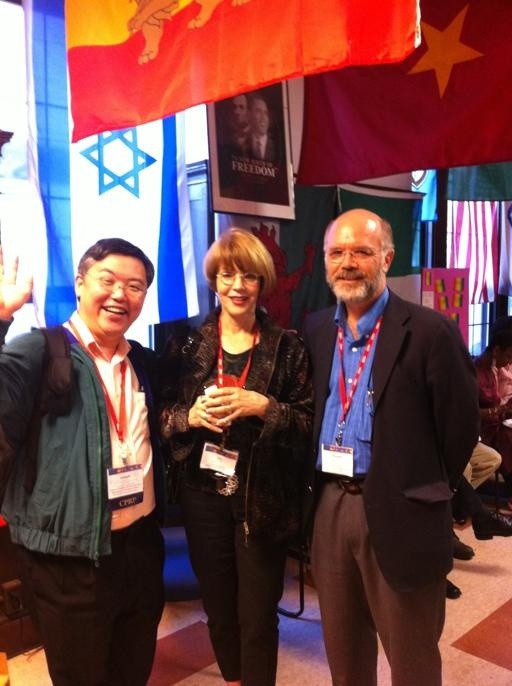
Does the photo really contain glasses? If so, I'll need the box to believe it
[329,248,374,264]
[96,272,143,299]
[217,271,258,285]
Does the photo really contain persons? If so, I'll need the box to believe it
[0,237,174,686]
[222,94,252,159]
[299,207,478,685]
[247,93,278,164]
[157,227,316,685]
[453,345,512,560]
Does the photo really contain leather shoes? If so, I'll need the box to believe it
[446,579,460,599]
[474,512,512,540]
[453,533,474,560]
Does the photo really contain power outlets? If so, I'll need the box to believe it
[0,578,25,617]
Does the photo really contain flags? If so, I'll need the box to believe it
[21,0,188,351]
[61,0,424,146]
[292,0,512,187]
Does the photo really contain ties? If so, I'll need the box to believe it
[254,141,262,160]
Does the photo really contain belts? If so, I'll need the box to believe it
[338,480,359,495]
